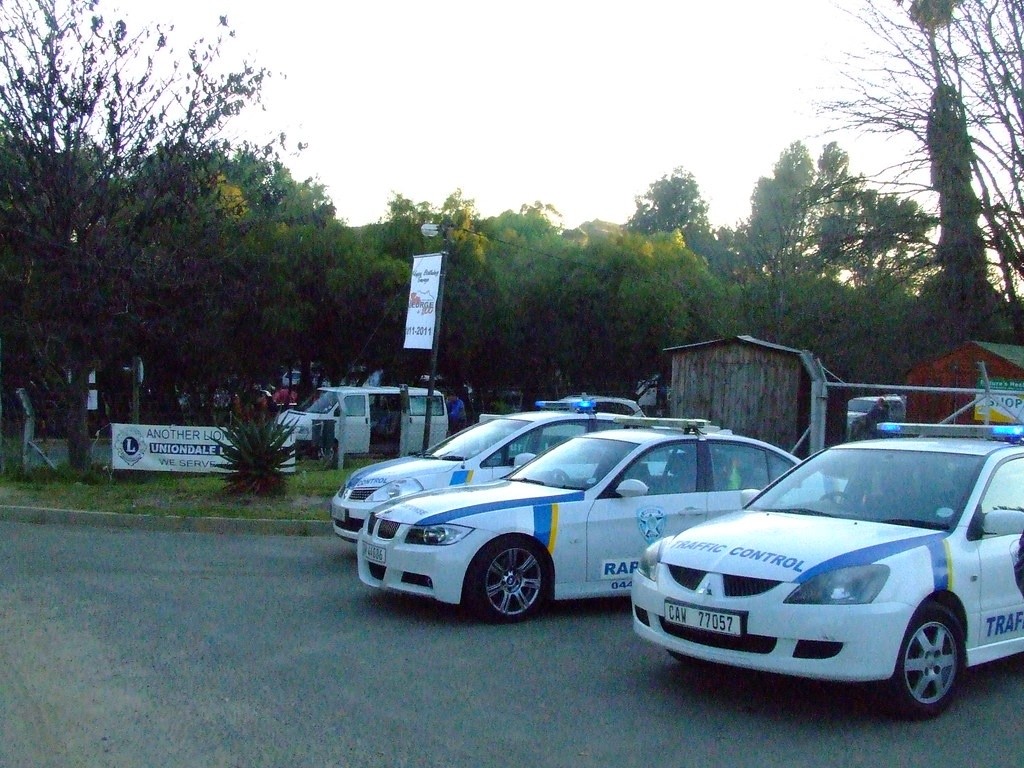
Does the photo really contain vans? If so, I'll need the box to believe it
[279,386,449,458]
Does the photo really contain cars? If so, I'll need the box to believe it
[357,418,804,624]
[624,415,1024,716]
[332,413,632,547]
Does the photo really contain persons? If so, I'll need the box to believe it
[864,397,885,440]
[445,391,467,436]
[240,389,279,428]
[874,403,893,439]
[250,381,273,416]
